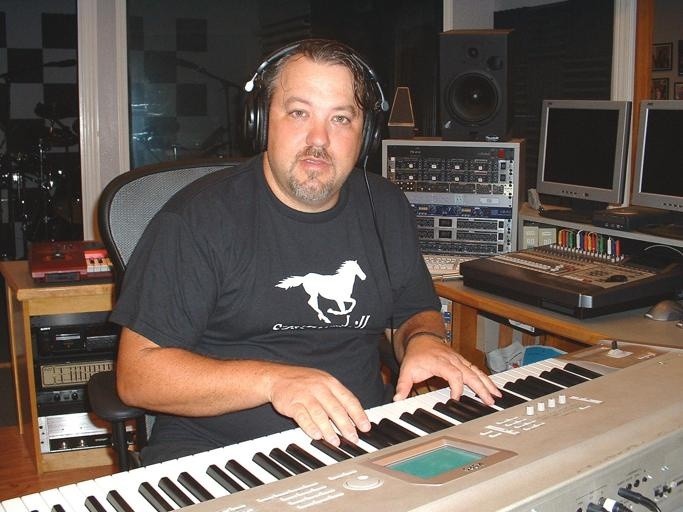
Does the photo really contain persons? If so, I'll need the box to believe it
[108,39,502,466]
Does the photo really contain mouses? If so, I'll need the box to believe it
[644,299,683,321]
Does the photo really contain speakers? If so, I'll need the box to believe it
[437,29,513,141]
[387,86,419,138]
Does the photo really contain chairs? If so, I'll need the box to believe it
[85,154,269,475]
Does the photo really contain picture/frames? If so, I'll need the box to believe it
[652,42,673,100]
[674,81,683,99]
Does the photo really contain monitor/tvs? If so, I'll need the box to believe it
[536,99,633,224]
[631,99,683,240]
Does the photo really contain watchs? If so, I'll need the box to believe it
[407,332,448,344]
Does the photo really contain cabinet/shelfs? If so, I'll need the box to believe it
[2,258,140,482]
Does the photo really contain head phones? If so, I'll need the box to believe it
[240,38,390,165]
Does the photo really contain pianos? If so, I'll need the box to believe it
[0,343,683,511]
